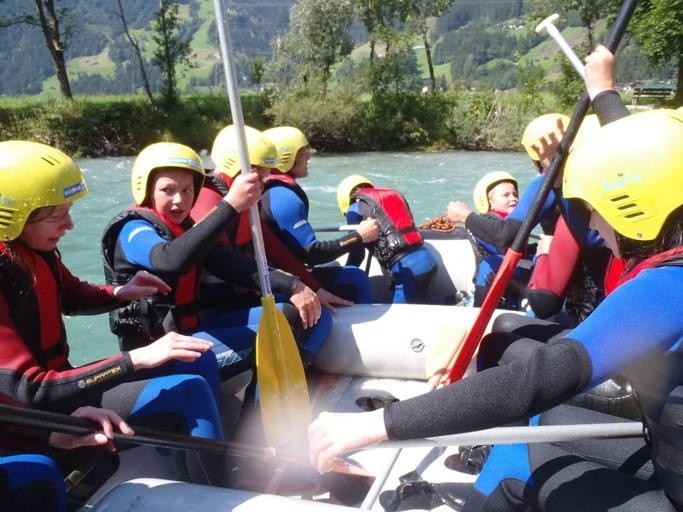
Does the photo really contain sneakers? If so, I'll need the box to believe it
[438,483,488,512]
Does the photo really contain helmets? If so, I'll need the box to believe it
[261,126,309,173]
[521,113,571,161]
[132,142,206,206]
[562,108,683,242]
[0,139,88,241]
[211,124,278,178]
[336,176,373,214]
[474,172,518,213]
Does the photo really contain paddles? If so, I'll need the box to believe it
[214,0,313,450]
[441,0,637,387]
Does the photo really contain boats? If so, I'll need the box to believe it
[62,220,548,511]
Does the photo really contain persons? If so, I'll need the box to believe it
[0,137,229,487]
[445,44,652,467]
[190,124,354,370]
[335,174,437,304]
[305,106,683,512]
[0,404,135,512]
[97,139,302,420]
[261,125,381,304]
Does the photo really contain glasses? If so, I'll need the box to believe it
[41,211,69,222]
